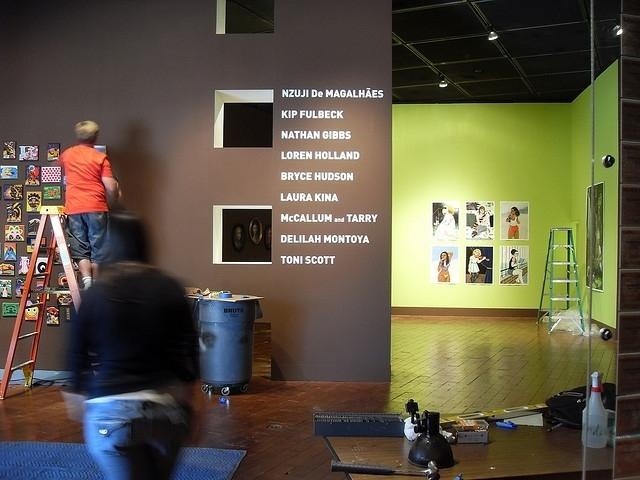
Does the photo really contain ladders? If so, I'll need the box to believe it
[0,205,85,401]
[536,227,586,336]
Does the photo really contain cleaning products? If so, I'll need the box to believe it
[581,372,608,450]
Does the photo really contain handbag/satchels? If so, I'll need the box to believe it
[542,382,616,423]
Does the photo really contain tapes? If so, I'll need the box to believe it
[219,292,233,299]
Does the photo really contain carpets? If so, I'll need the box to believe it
[0,440,249,480]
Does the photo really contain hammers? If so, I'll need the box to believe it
[329,459,440,480]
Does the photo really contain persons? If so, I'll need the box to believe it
[468,248,490,282]
[252,223,260,243]
[506,206,521,239]
[507,249,524,285]
[437,251,451,283]
[56,119,123,290]
[471,204,491,239]
[66,211,209,480]
[435,205,458,234]
[234,227,243,250]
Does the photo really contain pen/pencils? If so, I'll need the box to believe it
[547,422,565,432]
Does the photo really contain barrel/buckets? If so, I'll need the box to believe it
[198,299,253,388]
